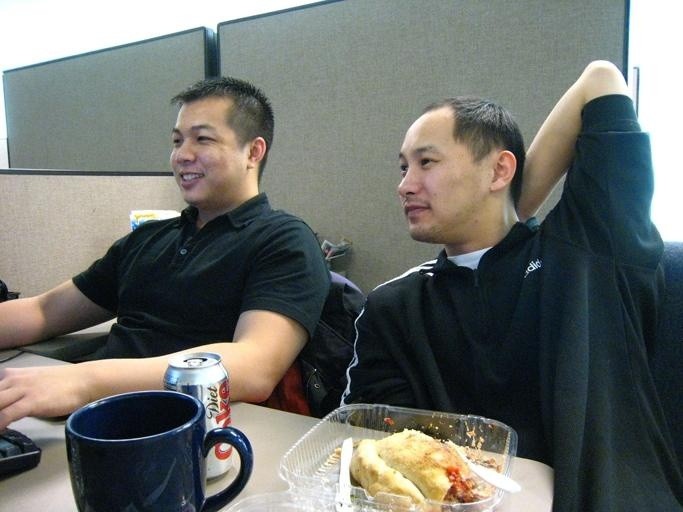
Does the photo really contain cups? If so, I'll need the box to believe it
[64,390,255,512]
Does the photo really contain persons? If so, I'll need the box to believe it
[340,59,682,512]
[0,75,332,437]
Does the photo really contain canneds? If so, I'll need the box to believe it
[163,351,234,484]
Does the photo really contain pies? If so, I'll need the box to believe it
[350,429,496,512]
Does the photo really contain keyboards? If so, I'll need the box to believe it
[0,425,43,479]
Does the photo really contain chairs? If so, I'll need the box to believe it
[660,240,683,330]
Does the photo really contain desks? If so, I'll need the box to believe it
[0,353,555,512]
